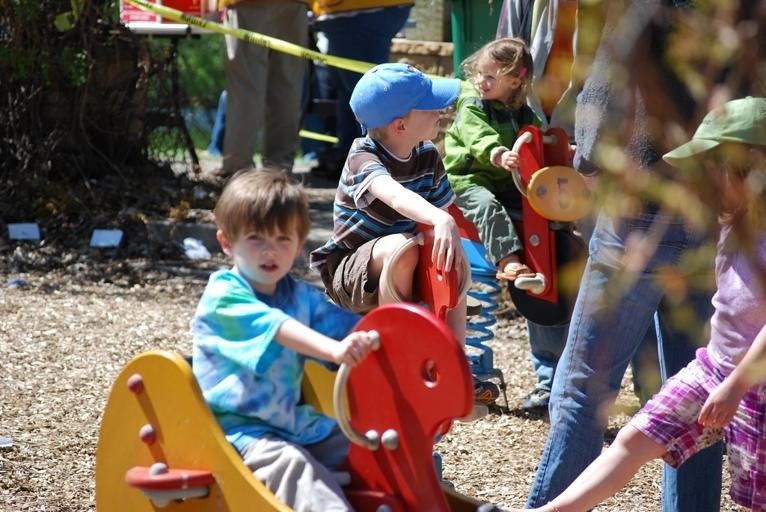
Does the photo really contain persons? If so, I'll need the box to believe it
[194,166,370,512]
[202,1,310,183]
[476,97,766,512]
[304,0,415,188]
[311,63,498,410]
[446,39,556,280]
[496,0,610,140]
[527,0,766,510]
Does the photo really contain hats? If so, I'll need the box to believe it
[661,95,766,168]
[349,63,461,137]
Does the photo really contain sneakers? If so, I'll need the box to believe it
[472,374,499,403]
[518,388,550,417]
